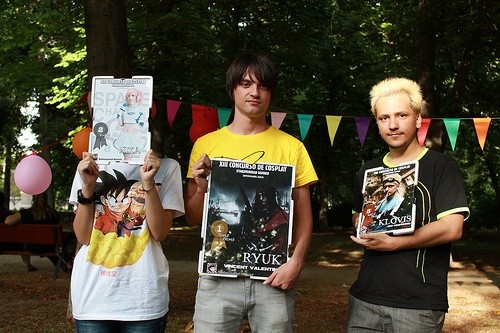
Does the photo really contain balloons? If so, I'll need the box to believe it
[189,115,214,143]
[72,127,92,159]
[14,155,52,195]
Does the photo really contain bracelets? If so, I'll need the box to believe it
[140,184,155,192]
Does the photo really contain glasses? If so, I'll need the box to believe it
[381,178,400,185]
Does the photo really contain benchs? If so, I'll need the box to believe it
[0,223,72,281]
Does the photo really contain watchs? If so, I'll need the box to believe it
[77,189,97,205]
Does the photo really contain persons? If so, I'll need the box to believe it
[113,88,149,160]
[345,77,470,333]
[184,52,319,333]
[5,193,73,273]
[241,178,289,277]
[0,192,39,271]
[372,173,412,230]
[69,75,186,333]
